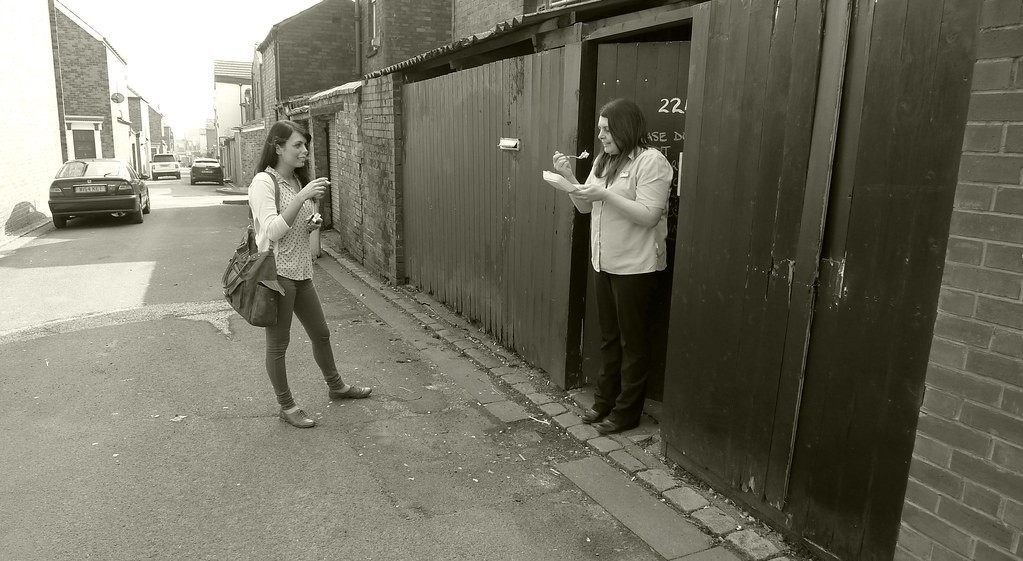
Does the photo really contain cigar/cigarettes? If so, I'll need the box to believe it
[325,180,330,185]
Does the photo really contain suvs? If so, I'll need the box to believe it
[148,153,182,180]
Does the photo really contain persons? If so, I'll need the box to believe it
[248,120,372,428]
[553,99,673,435]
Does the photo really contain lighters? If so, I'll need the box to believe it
[307,215,314,224]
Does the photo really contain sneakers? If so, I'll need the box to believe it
[329,385,372,399]
[279,408,314,428]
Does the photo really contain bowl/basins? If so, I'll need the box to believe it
[543,169,590,194]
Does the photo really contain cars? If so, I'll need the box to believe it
[48,158,151,231]
[188,158,224,186]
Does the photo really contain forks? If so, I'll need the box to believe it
[570,150,590,159]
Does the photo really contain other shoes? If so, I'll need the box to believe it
[582,408,606,424]
[596,420,625,434]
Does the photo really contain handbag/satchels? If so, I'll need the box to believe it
[221,224,285,327]
